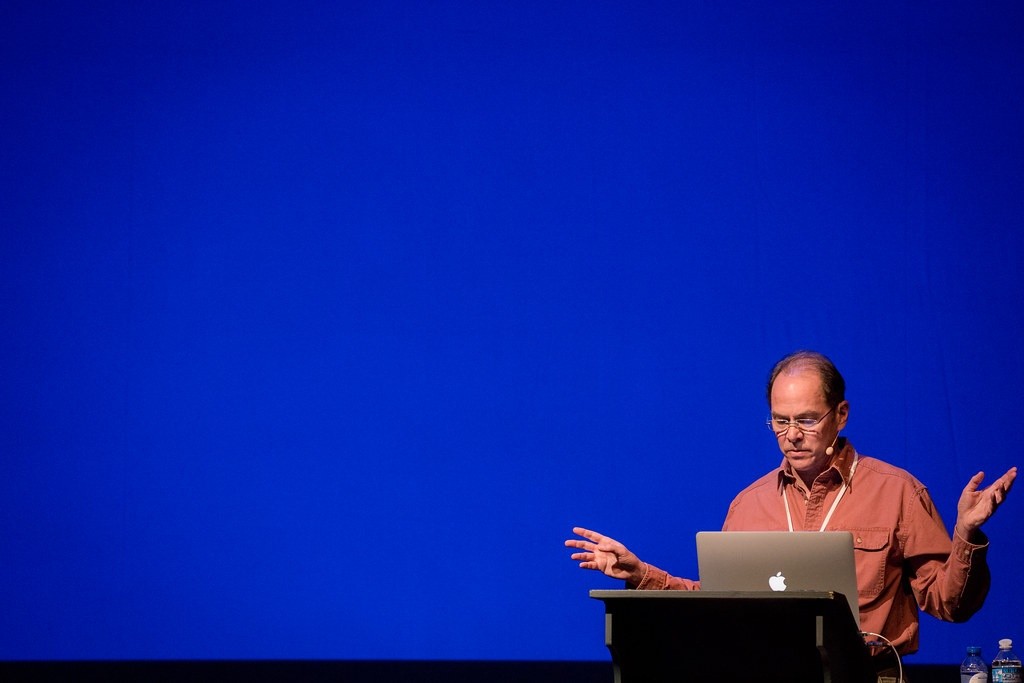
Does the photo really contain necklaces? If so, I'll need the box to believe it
[779,450,860,533]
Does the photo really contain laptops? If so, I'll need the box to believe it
[697,531,862,633]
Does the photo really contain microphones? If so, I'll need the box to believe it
[826,432,840,456]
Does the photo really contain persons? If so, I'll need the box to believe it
[565,349,1018,682]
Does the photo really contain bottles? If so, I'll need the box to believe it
[992,639,1021,683]
[960,646,988,683]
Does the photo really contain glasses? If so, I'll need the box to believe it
[766,405,835,432]
